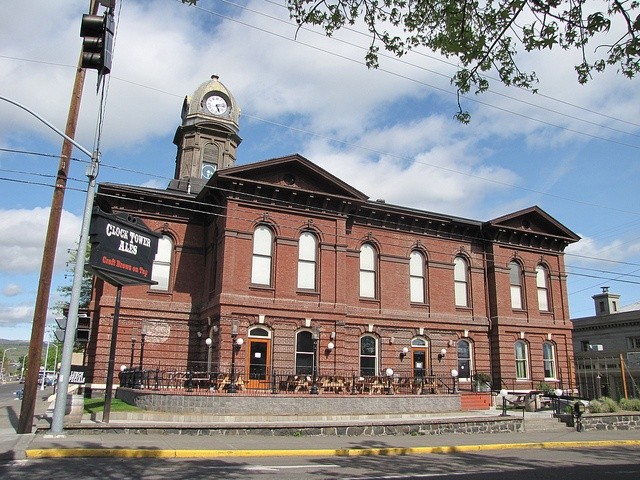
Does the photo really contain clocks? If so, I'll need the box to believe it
[202,161,217,179]
[205,93,229,115]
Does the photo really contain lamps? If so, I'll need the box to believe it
[327,343,335,352]
[206,338,214,348]
[236,338,244,348]
[440,349,448,358]
[402,347,409,357]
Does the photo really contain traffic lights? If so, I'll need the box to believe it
[54,319,67,342]
[78,13,113,74]
[76,317,92,341]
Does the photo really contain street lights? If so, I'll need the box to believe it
[41,334,50,391]
[230,319,239,385]
[311,322,319,388]
[139,321,148,370]
[130,327,138,368]
[20,354,29,380]
[52,343,58,387]
[1,348,16,380]
